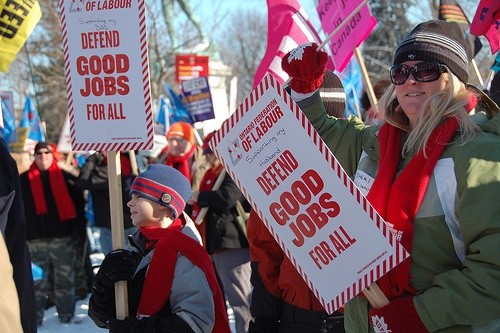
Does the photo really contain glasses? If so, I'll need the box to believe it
[390,61,447,85]
[36,150,50,155]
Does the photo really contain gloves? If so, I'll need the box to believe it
[96,248,139,287]
[281,42,329,94]
[368,295,429,333]
[108,317,132,333]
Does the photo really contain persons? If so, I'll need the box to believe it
[0,19,500,333]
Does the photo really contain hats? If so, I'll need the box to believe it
[201,131,217,156]
[166,121,195,145]
[34,141,52,153]
[129,164,192,219]
[393,19,470,86]
[285,70,346,117]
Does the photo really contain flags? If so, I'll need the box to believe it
[163,80,193,125]
[0,0,42,73]
[20,96,42,142]
[0,99,16,133]
[0,127,13,152]
[439,0,500,73]
[157,100,178,127]
[333,41,367,119]
[252,0,338,88]
